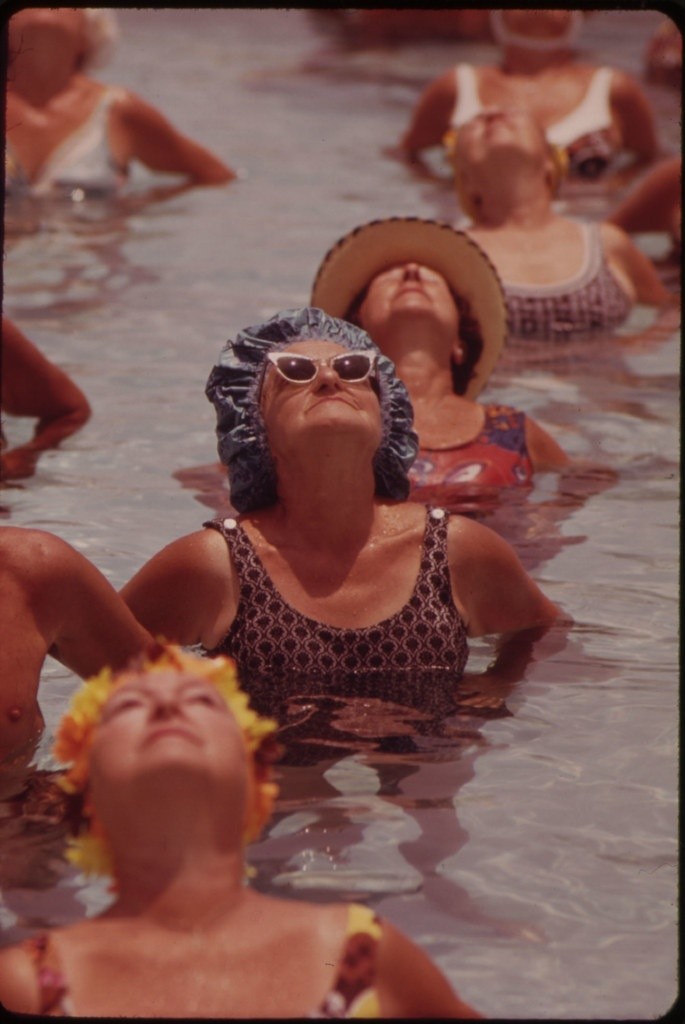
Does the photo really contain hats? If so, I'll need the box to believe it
[205,308,419,514]
[310,217,509,402]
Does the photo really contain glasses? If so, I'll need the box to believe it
[257,349,376,408]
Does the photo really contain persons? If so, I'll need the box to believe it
[117,307,559,668]
[5,8,217,198]
[0,642,489,1019]
[0,526,158,762]
[608,155,681,243]
[308,216,565,485]
[441,108,666,337]
[401,9,658,183]
[1,314,93,451]
